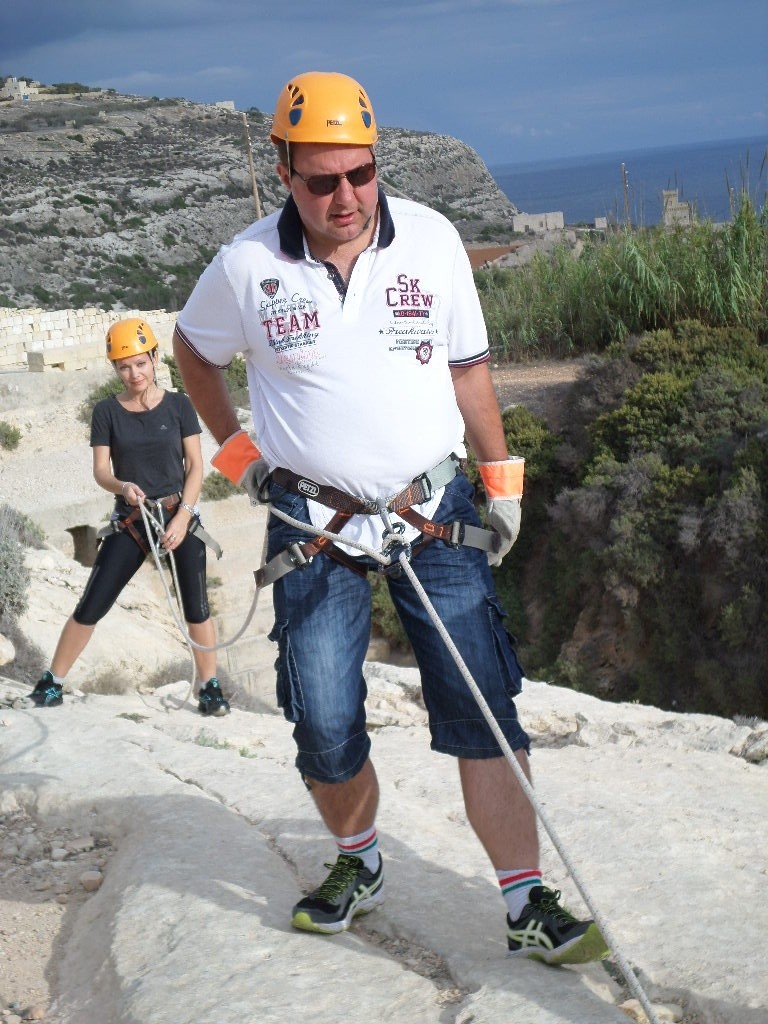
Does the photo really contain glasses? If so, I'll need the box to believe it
[290,149,376,195]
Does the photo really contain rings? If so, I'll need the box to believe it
[172,534,177,539]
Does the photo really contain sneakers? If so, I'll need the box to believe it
[289,851,385,935]
[12,671,63,708]
[198,677,230,716]
[504,886,611,966]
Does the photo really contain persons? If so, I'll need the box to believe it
[173,70,609,965]
[23,319,230,717]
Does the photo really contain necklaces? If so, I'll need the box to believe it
[129,387,159,411]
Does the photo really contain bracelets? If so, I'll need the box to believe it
[122,482,134,499]
[179,503,196,518]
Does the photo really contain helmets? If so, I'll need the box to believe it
[105,318,157,361]
[270,72,378,144]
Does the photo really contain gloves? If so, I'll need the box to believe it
[210,429,270,505]
[477,455,525,567]
